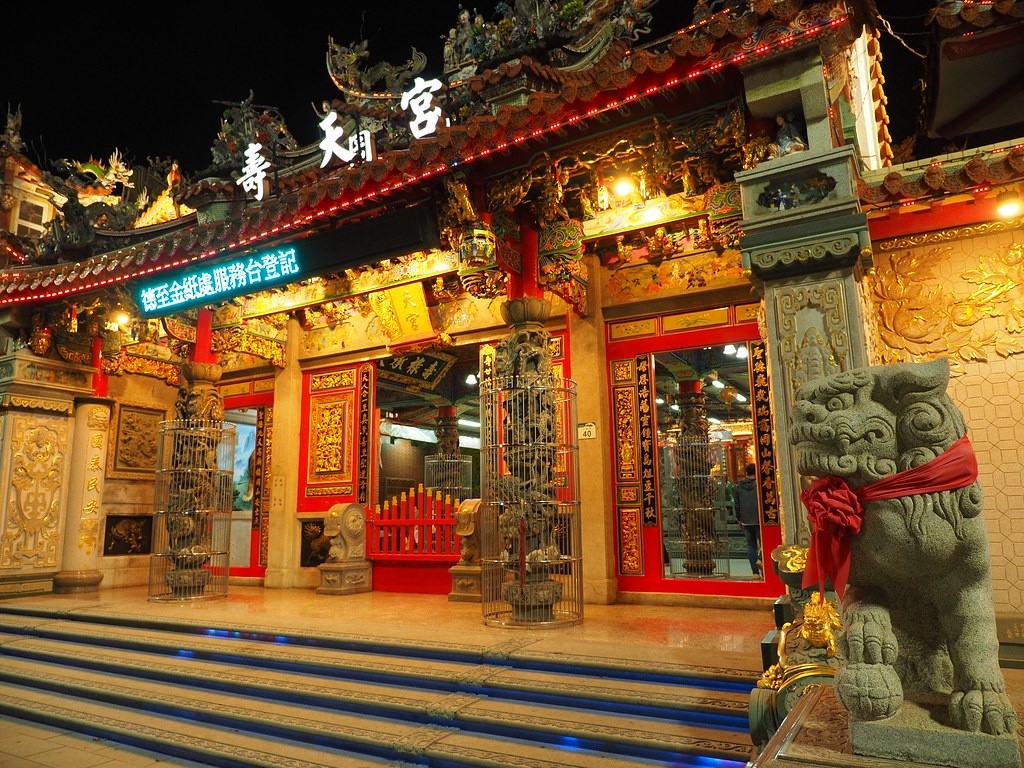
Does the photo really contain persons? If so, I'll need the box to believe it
[733,463,762,579]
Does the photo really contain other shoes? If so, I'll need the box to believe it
[756,560,764,575]
[752,574,761,580]
